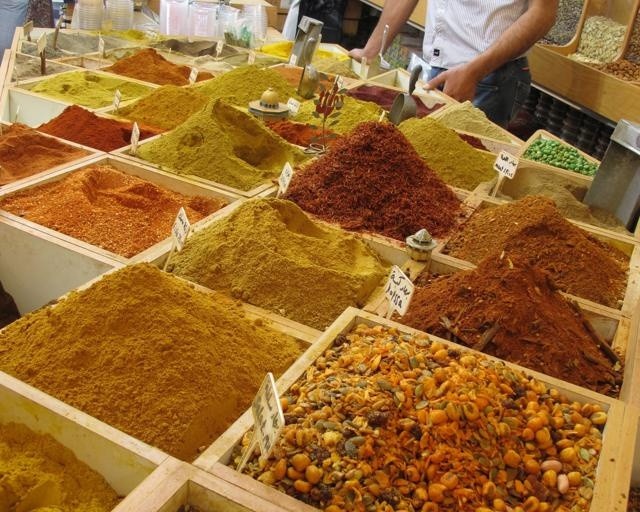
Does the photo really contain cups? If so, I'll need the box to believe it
[76,1,134,31]
[241,5,267,41]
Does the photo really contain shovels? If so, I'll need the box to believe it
[367,25,389,78]
[297,38,318,99]
[389,65,422,126]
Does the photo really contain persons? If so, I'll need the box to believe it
[347,0,559,129]
[0,0,29,66]
